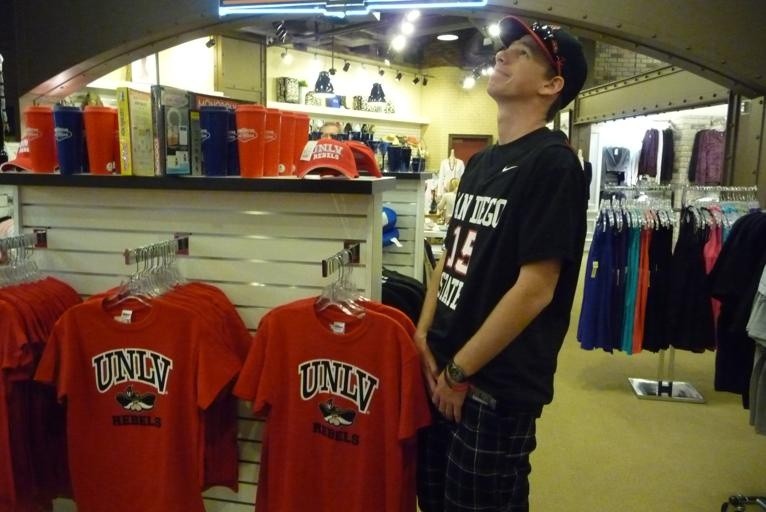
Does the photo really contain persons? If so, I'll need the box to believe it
[318,121,343,142]
[413,15,589,511]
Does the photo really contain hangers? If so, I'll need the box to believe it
[0,234,49,296]
[314,252,368,325]
[101,241,182,322]
[597,184,760,232]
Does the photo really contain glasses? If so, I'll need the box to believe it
[527,19,562,70]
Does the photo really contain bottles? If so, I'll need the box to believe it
[225,108,240,176]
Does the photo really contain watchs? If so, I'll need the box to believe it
[447,358,468,384]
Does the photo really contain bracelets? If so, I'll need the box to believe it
[443,367,473,391]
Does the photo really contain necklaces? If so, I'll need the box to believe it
[448,158,456,171]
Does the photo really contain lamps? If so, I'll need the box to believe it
[313,70,335,100]
[343,63,350,72]
[377,69,385,77]
[395,71,401,81]
[206,39,217,48]
[422,76,428,86]
[412,77,420,85]
[367,82,389,107]
[473,70,481,79]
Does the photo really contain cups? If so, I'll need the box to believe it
[84,105,115,175]
[277,109,296,177]
[264,108,283,176]
[51,105,85,175]
[294,112,311,176]
[22,106,57,173]
[236,104,266,178]
[198,104,229,177]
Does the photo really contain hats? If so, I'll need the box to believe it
[295,138,359,182]
[498,14,587,109]
[339,140,382,179]
[1,133,57,175]
